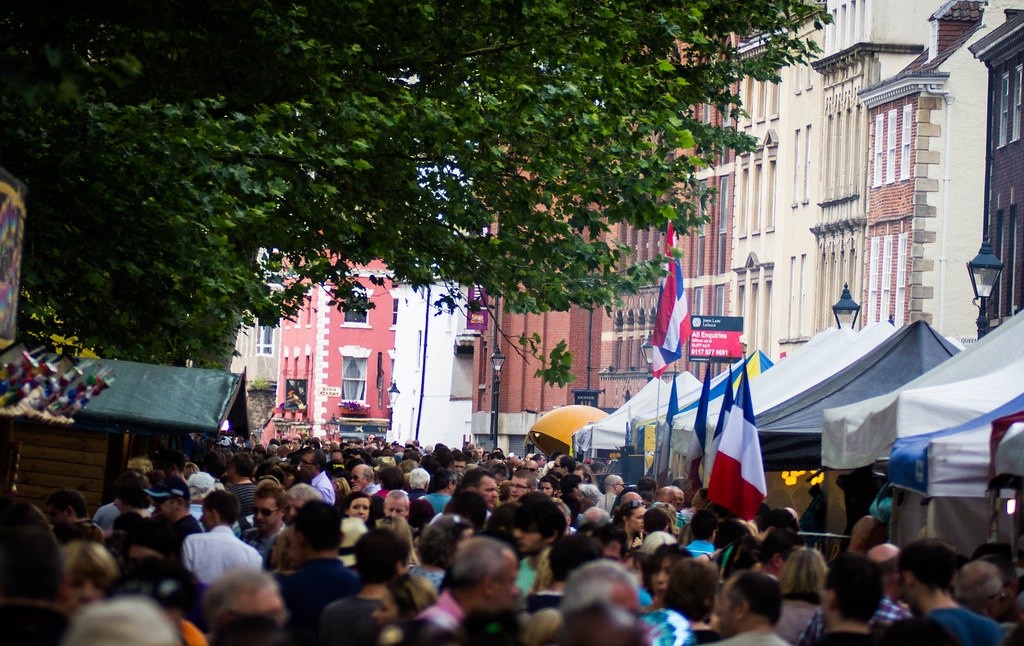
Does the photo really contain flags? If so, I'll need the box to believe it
[707,362,768,521]
[687,364,710,496]
[656,372,681,487]
[708,369,735,479]
[652,219,694,380]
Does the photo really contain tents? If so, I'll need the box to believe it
[572,308,1024,556]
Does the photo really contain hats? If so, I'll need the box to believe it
[374,457,396,472]
[144,476,191,500]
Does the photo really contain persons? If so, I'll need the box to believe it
[0,434,1024,646]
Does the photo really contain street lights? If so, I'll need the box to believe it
[387,381,400,440]
[966,236,1006,341]
[641,332,653,384]
[490,346,506,450]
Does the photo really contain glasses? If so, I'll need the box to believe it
[618,483,627,488]
[554,462,561,468]
[511,484,531,489]
[351,475,368,480]
[632,501,645,508]
[253,508,280,517]
[524,468,536,472]
